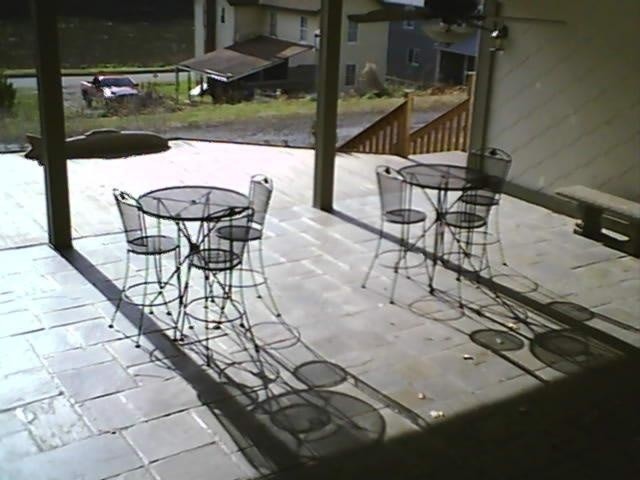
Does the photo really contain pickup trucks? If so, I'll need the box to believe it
[81,75,140,107]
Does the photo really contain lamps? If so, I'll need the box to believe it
[417,18,478,48]
[488,25,509,40]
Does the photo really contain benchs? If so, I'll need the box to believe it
[553,184,640,258]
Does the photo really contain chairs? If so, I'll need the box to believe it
[106,173,282,369]
[360,147,512,311]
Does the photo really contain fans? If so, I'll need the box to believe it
[344,0,570,40]
